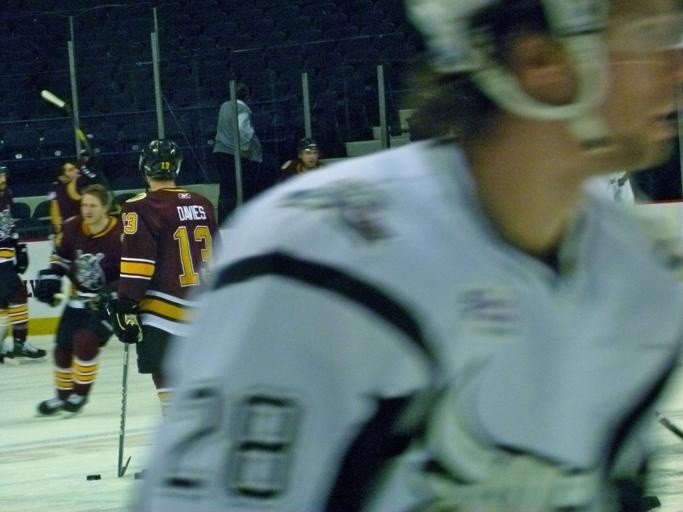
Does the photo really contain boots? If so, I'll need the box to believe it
[63,393,89,413]
[36,396,66,416]
[13,339,47,360]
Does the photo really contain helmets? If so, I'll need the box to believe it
[137,137,184,182]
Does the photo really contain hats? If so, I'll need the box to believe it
[296,136,321,149]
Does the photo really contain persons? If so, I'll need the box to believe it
[0,165,52,364]
[50,154,122,251]
[112,137,223,479]
[593,162,644,206]
[33,184,130,421]
[126,2,682,511]
[280,130,330,180]
[212,75,263,210]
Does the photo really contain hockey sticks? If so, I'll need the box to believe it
[118,343,130,478]
[41,89,124,214]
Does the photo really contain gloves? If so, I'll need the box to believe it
[32,266,62,306]
[105,298,144,343]
